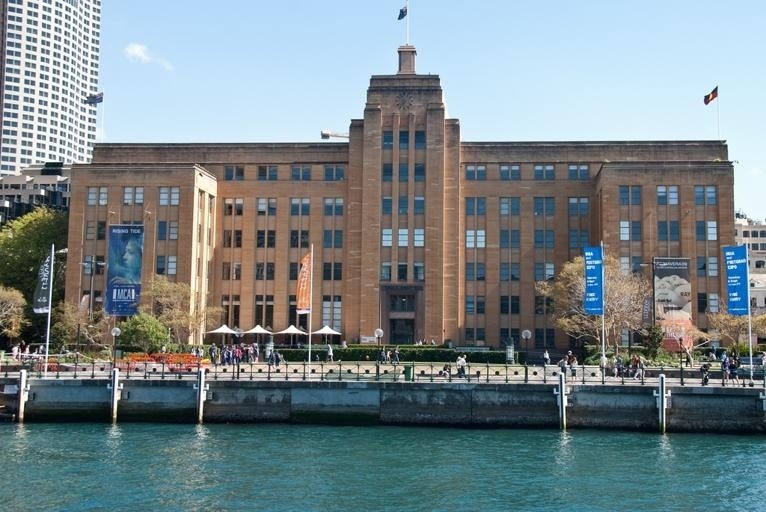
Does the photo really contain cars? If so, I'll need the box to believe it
[737,350,765,366]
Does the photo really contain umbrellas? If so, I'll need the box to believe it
[276,325,309,345]
[202,325,238,345]
[310,325,342,344]
[241,324,275,344]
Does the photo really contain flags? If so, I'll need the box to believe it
[703,87,717,105]
[397,5,406,20]
[83,92,102,104]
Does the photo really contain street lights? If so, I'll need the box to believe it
[674,332,686,386]
[78,255,107,331]
[234,328,245,379]
[108,328,122,369]
[521,328,532,383]
[372,327,383,380]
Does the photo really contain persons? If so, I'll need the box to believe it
[109,238,140,285]
[461,355,467,378]
[558,350,646,380]
[455,356,462,378]
[190,343,283,369]
[443,364,449,379]
[386,350,391,364]
[380,345,386,363]
[10,340,66,361]
[700,344,765,386]
[393,344,399,364]
[413,338,435,346]
[324,344,333,363]
